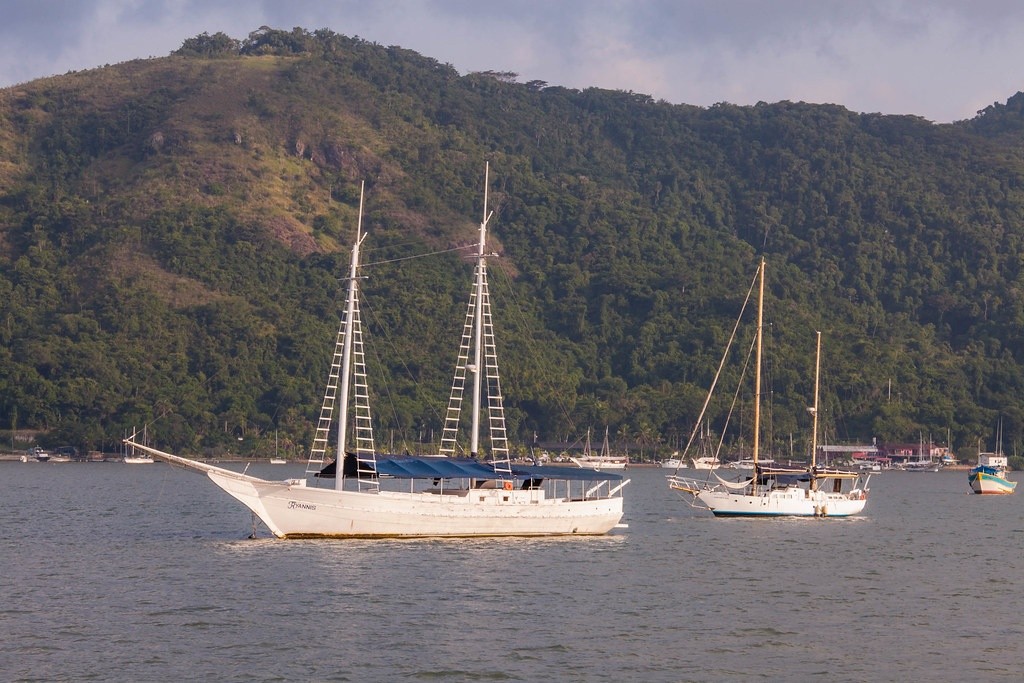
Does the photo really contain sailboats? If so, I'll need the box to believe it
[905,430,955,473]
[725,394,775,469]
[123,425,154,464]
[123,160,631,540]
[664,255,871,518]
[690,418,721,470]
[269,429,287,464]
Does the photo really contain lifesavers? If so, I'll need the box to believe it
[861,494,865,500]
[505,482,512,490]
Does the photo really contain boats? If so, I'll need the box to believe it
[660,458,688,469]
[859,460,882,473]
[967,417,1018,494]
[568,424,627,469]
[88,450,104,462]
[28,445,51,462]
